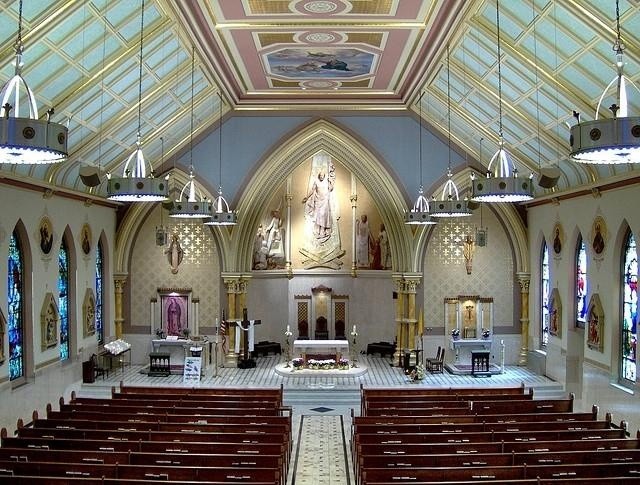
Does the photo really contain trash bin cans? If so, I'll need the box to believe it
[83,360,95,383]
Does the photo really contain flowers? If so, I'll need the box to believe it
[481,329,490,337]
[291,357,349,367]
[154,328,164,335]
[451,328,460,336]
[183,328,191,334]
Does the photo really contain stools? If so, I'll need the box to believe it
[471,350,491,377]
[148,352,171,377]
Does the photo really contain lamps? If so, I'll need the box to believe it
[203,90,238,226]
[471,0,535,203]
[105,0,169,202]
[569,0,640,165]
[0,0,68,165]
[403,90,439,225]
[429,46,473,218]
[168,46,214,218]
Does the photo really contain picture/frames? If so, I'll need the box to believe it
[161,295,188,339]
[464,327,476,338]
[293,365,349,370]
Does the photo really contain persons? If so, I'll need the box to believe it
[302,172,333,238]
[164,236,182,267]
[355,214,388,270]
[167,298,181,336]
[253,209,285,270]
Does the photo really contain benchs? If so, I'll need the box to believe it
[251,341,281,357]
[367,342,397,359]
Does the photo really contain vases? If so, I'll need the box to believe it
[483,337,489,339]
[157,334,161,340]
[185,334,188,340]
[452,335,459,341]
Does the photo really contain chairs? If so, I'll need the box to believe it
[430,348,445,374]
[335,320,346,340]
[426,346,441,371]
[315,316,328,340]
[350,384,640,485]
[297,320,309,340]
[0,381,292,485]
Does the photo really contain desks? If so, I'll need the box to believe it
[294,340,349,368]
